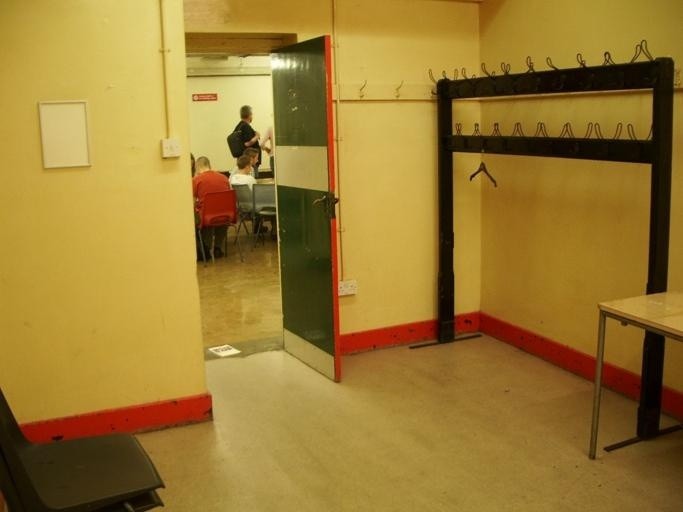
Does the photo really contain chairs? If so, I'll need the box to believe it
[0,387,166,512]
[231,184,253,246]
[253,184,276,248]
[197,190,244,267]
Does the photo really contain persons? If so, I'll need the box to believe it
[229,147,268,234]
[191,153,196,177]
[192,156,230,260]
[229,157,277,240]
[227,105,261,179]
[261,127,274,178]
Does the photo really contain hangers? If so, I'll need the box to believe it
[469,150,496,187]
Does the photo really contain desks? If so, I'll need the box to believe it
[589,291,683,459]
[255,177,275,184]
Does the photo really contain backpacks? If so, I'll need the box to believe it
[227,131,245,157]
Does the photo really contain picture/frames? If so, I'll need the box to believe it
[38,100,92,170]
